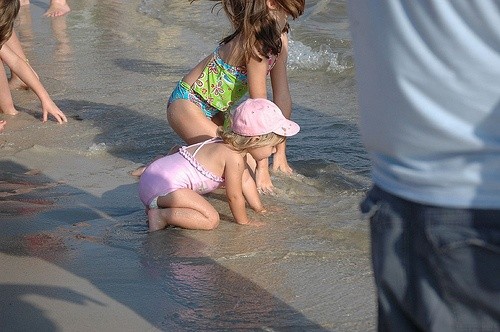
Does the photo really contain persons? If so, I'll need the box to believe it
[346,1,500,332]
[1,0,72,128]
[140,99,299,236]
[168,0,304,197]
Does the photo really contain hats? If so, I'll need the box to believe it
[231,97,300,137]
